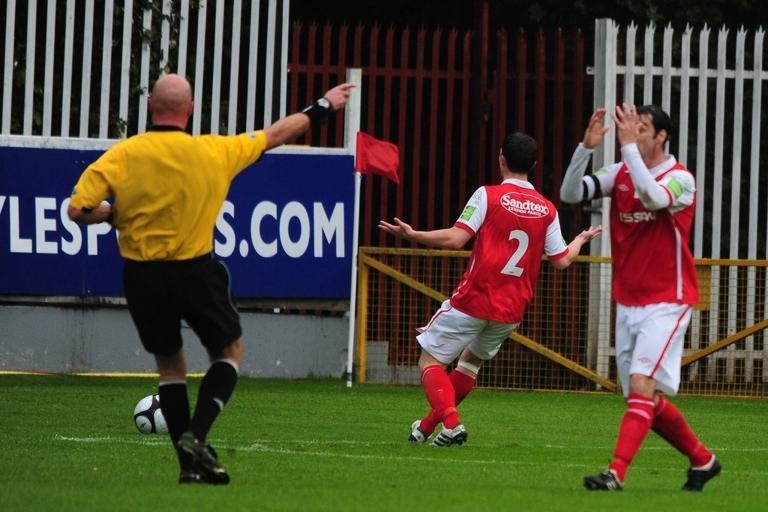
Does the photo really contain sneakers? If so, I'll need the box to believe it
[681,456,721,491]
[408,419,426,442]
[177,430,229,484]
[582,471,622,490]
[431,423,467,446]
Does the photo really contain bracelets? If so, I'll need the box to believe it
[300,97,335,127]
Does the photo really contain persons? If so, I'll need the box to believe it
[68,74,358,486]
[376,133,602,449]
[560,101,721,491]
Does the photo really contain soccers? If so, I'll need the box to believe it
[133,394,168,435]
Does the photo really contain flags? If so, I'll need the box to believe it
[355,130,400,186]
